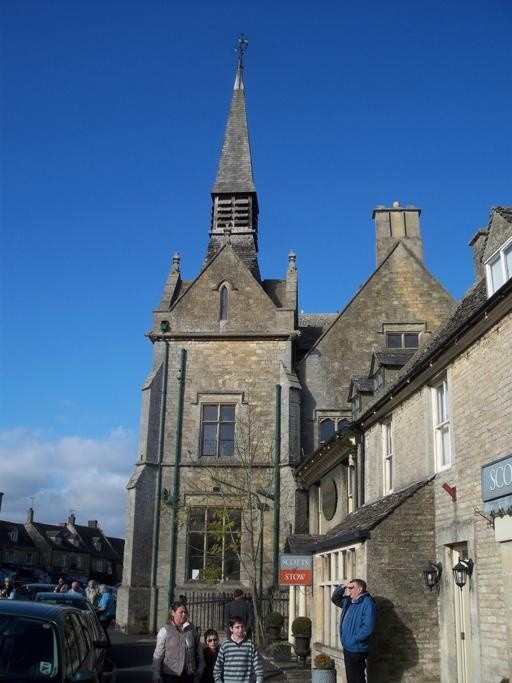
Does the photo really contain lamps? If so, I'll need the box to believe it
[452,556,474,592]
[423,559,442,591]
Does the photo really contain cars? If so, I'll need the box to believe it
[0,567,122,682]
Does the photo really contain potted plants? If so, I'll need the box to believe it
[266,611,312,663]
[311,653,336,683]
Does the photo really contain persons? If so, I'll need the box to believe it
[85,580,100,608]
[331,579,376,683]
[97,585,115,647]
[150,601,207,683]
[195,629,224,683]
[0,577,18,598]
[68,581,85,596]
[211,617,264,683]
[53,578,68,593]
[8,583,32,600]
[224,588,255,639]
